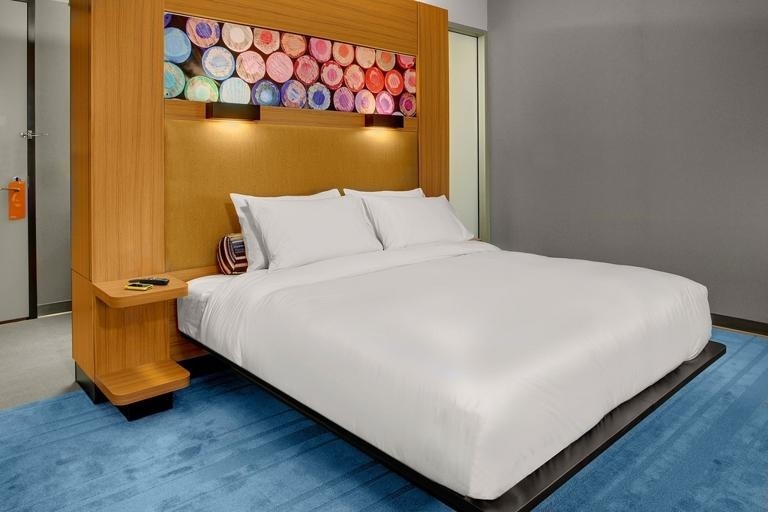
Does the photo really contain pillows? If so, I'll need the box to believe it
[361,194,476,251]
[226,186,342,274]
[344,184,426,256]
[243,194,386,274]
[214,232,248,275]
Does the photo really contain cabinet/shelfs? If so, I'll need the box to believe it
[91,275,190,407]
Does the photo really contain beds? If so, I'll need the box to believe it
[172,244,728,511]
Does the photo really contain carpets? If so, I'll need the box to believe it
[0,319,768,511]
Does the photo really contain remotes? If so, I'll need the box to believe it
[128,277,169,285]
